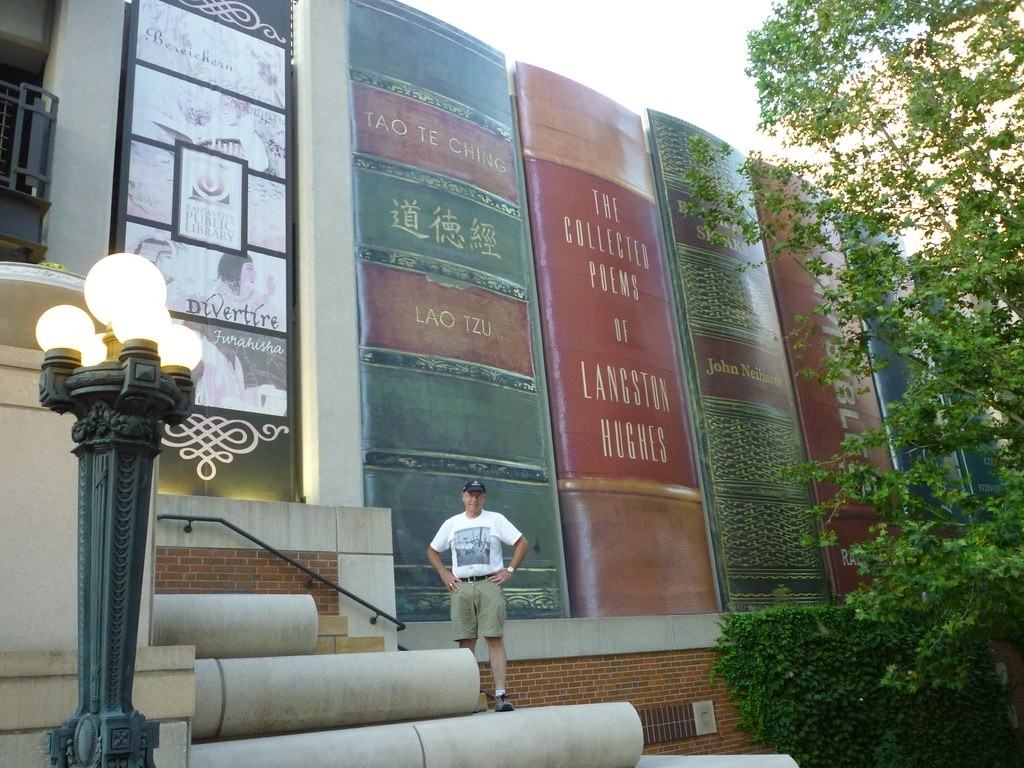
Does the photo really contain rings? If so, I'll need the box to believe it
[449,584,452,587]
[497,577,501,581]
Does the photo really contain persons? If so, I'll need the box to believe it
[428,480,528,712]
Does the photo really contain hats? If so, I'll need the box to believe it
[462,479,486,493]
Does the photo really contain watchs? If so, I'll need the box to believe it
[508,567,514,573]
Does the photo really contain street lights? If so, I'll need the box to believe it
[36,253,204,768]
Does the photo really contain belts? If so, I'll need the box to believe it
[455,575,494,582]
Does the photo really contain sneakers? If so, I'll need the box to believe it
[495,694,515,711]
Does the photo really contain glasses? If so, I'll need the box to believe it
[465,492,485,499]
